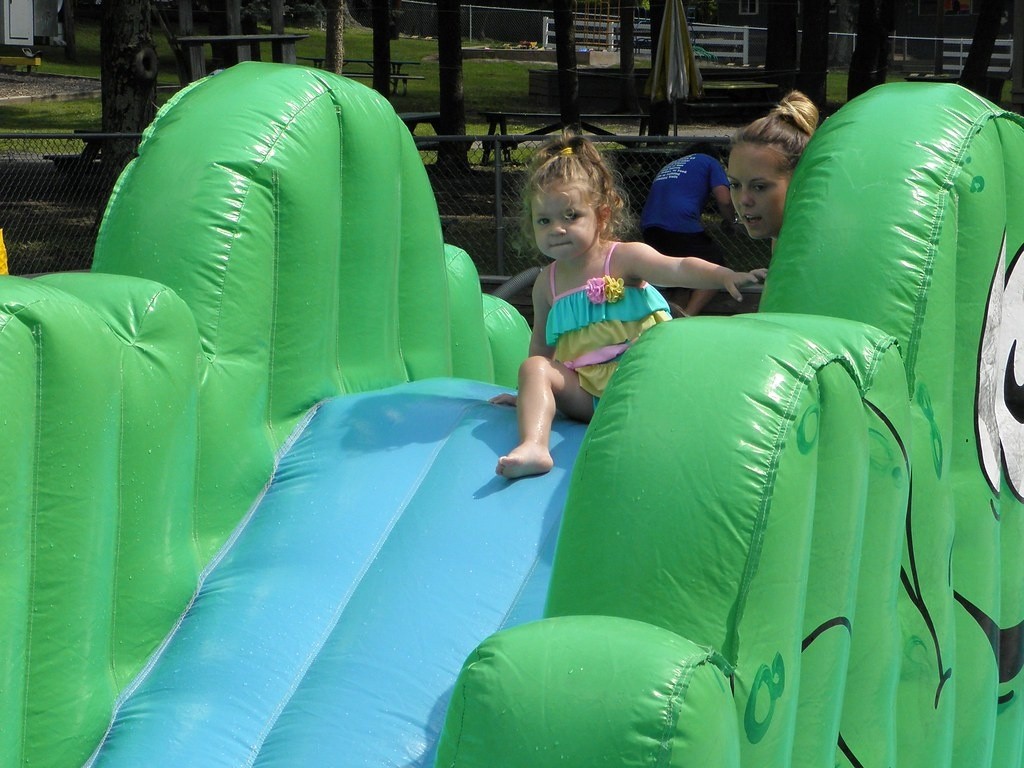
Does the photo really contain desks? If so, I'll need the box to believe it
[482,111,651,166]
[298,56,420,94]
[74,130,102,159]
[400,113,440,136]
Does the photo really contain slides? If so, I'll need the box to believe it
[74,377,594,767]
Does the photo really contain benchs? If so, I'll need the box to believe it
[44,153,100,163]
[340,71,425,97]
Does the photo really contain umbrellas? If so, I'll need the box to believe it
[642,0,703,136]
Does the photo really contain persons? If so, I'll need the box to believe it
[679,89,818,318]
[488,136,769,479]
[642,145,732,316]
